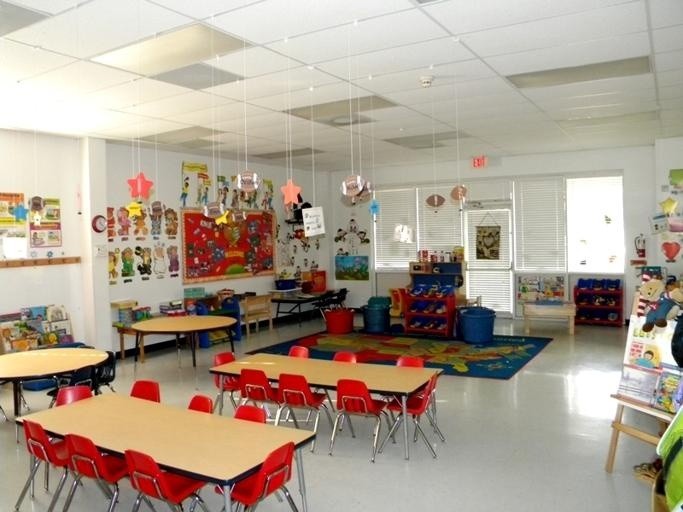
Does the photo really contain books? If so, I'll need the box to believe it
[0,302,74,355]
[159,300,189,316]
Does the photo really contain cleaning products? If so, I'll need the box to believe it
[454,245,465,261]
[633,230,645,259]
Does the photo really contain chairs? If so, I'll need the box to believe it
[61,435,153,512]
[214,442,299,511]
[14,419,101,511]
[275,373,338,453]
[330,378,397,463]
[233,404,268,424]
[1,286,452,348]
[287,345,308,358]
[187,394,214,414]
[209,351,241,412]
[238,368,283,423]
[321,351,357,413]
[378,371,445,459]
[380,355,425,424]
[131,380,161,403]
[56,386,92,407]
[124,449,208,512]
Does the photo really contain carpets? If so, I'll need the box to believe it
[247,328,554,381]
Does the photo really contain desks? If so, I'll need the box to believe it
[210,353,444,459]
[0,348,107,444]
[523,301,574,336]
[15,390,318,511]
[130,315,237,369]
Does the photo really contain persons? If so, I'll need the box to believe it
[180,175,273,212]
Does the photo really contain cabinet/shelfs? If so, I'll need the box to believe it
[405,261,467,306]
[574,287,623,328]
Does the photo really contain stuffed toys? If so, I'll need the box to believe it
[638,279,671,330]
[662,280,683,320]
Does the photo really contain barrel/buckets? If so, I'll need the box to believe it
[324,307,354,335]
[362,303,389,334]
[459,306,495,343]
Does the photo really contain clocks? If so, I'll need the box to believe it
[92,215,106,232]
